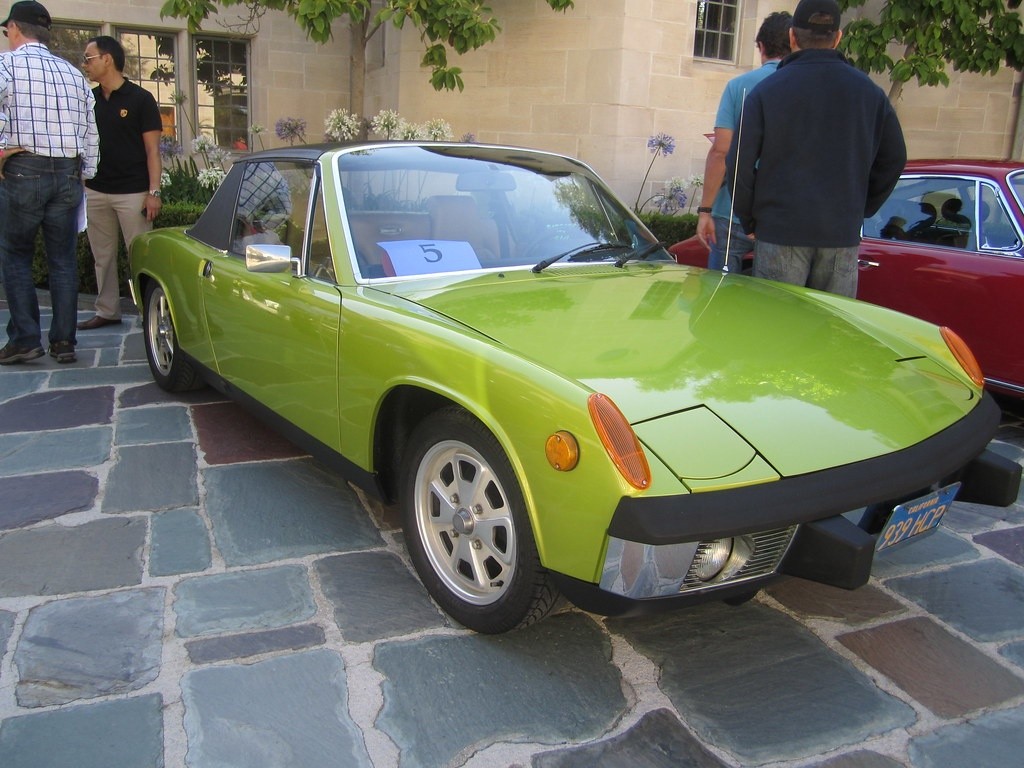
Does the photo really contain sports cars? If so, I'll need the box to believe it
[127,142,1022,637]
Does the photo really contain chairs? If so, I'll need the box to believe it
[880,196,938,245]
[347,211,433,265]
[427,196,500,261]
[288,194,337,282]
[953,197,986,250]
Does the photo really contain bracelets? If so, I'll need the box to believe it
[697,207,712,214]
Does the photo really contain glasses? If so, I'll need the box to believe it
[2,25,13,37]
[83,54,101,64]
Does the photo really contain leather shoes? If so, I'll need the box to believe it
[77,315,121,329]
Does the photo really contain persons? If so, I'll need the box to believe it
[77,36,163,331]
[0,0,100,364]
[880,191,972,249]
[697,0,907,299]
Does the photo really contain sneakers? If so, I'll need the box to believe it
[47,340,77,363]
[0,338,45,365]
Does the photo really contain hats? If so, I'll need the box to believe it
[0,0,52,30]
[792,0,841,31]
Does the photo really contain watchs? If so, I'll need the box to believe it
[149,190,161,196]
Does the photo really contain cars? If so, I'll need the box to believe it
[667,158,1024,400]
